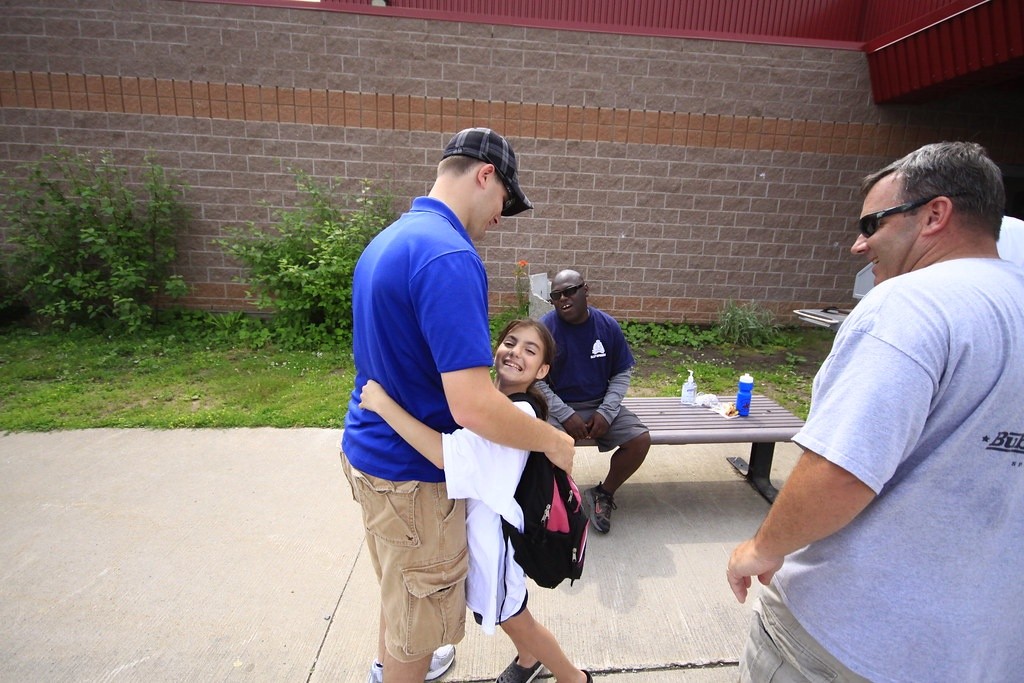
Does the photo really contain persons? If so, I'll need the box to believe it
[357,317,593,683]
[537,270,651,534]
[342,128,551,683]
[726,144,1023,683]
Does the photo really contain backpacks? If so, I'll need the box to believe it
[503,394,589,589]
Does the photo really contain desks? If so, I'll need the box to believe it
[793,308,856,331]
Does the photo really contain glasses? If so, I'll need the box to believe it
[482,153,516,209]
[550,283,585,300]
[860,191,975,238]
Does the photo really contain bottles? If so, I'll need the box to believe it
[735,373,754,416]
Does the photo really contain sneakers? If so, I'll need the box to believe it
[583,482,615,533]
[372,642,457,683]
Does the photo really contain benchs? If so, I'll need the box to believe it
[574,394,808,507]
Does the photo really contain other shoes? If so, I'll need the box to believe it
[494,656,544,683]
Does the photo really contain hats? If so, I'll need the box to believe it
[441,126,534,217]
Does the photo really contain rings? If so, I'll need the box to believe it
[726,567,731,574]
